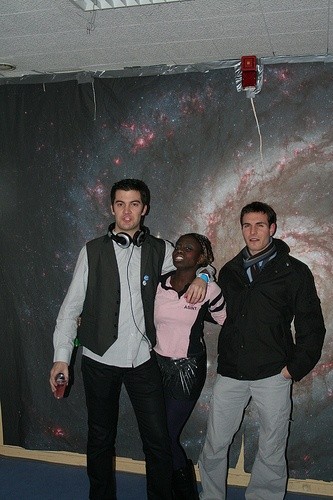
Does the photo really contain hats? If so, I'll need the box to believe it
[192,233,214,267]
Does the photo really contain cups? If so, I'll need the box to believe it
[53,374,66,399]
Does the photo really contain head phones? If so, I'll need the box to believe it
[108,222,147,249]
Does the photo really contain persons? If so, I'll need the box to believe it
[200,201,327,500]
[152,233,227,500]
[50,178,217,500]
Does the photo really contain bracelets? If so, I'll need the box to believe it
[196,273,210,285]
